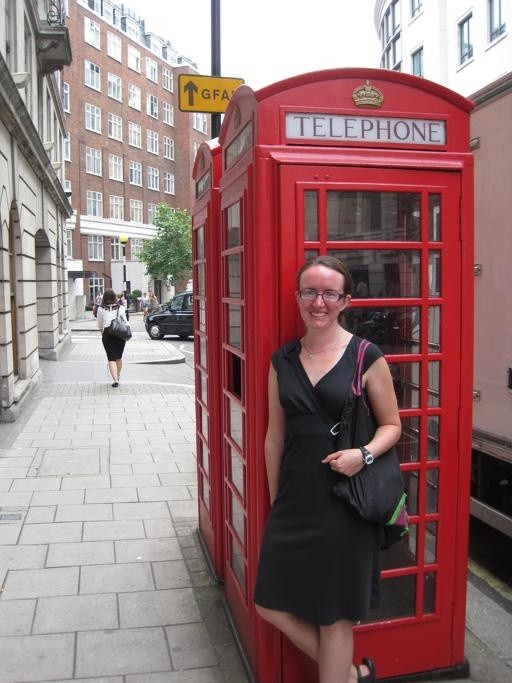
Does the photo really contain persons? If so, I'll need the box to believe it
[149,291,158,311]
[117,290,127,308]
[140,292,151,322]
[97,288,128,388]
[95,292,104,318]
[254,255,402,681]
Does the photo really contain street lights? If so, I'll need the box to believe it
[119,233,131,321]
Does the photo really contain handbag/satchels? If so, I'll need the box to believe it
[334,339,409,551]
[108,304,132,341]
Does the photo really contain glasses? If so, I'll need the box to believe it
[299,287,346,302]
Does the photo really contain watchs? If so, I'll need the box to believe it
[359,443,374,464]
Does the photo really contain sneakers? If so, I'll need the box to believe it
[112,382,119,387]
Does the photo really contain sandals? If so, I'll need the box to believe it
[353,657,376,683]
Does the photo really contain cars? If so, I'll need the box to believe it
[145,291,193,339]
